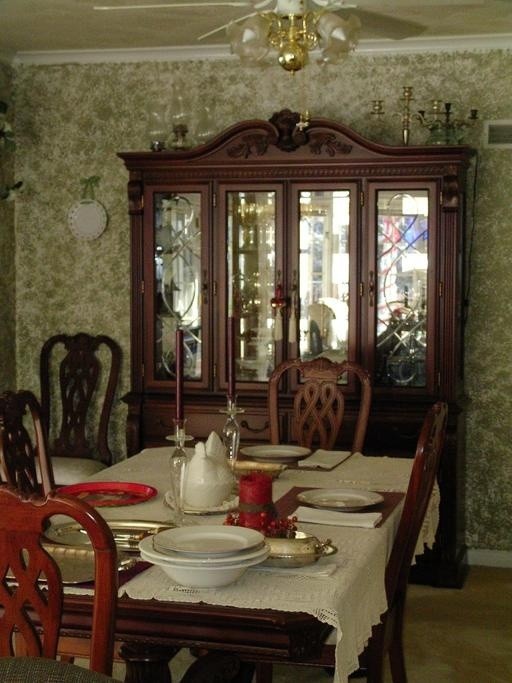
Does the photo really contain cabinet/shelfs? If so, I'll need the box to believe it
[117,391,469,589]
[116,107,478,405]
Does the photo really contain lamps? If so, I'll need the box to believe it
[226,1,362,132]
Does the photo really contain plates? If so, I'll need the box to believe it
[164,487,239,515]
[6,540,138,585]
[43,518,179,552]
[67,198,108,242]
[239,443,311,463]
[152,523,265,557]
[296,486,383,511]
[137,531,271,566]
[55,481,158,507]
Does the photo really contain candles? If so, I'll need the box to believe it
[176,330,184,430]
[228,318,236,405]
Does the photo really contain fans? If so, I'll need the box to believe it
[94,0,428,40]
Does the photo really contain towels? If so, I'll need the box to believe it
[298,448,350,470]
[286,504,383,529]
[248,561,337,577]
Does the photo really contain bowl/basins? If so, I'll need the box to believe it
[229,461,290,480]
[137,551,272,588]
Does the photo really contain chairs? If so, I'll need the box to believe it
[268,357,371,453]
[0,332,122,486]
[190,401,448,683]
[0,389,181,665]
[0,389,56,497]
[0,484,121,683]
